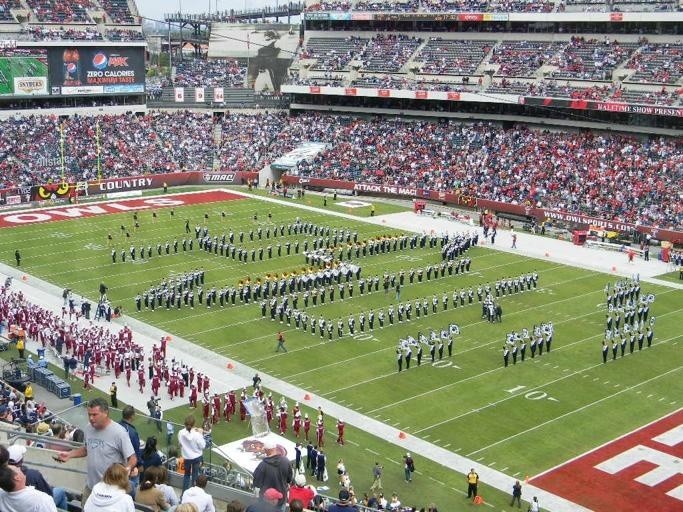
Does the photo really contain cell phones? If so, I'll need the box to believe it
[52,455,66,462]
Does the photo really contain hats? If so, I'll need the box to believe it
[7,445,27,464]
[339,489,350,502]
[261,438,277,449]
[263,488,283,500]
[37,422,50,434]
[294,474,306,487]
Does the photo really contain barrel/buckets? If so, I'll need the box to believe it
[72,393,82,406]
[37,348,45,357]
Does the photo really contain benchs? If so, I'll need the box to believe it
[50,484,155,512]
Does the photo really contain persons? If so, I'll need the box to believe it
[509,480,522,508]
[14,249,21,267]
[0,0,335,207]
[464,468,480,500]
[108,205,556,373]
[590,234,682,365]
[337,0,683,234]
[527,495,539,511]
[0,275,436,511]
